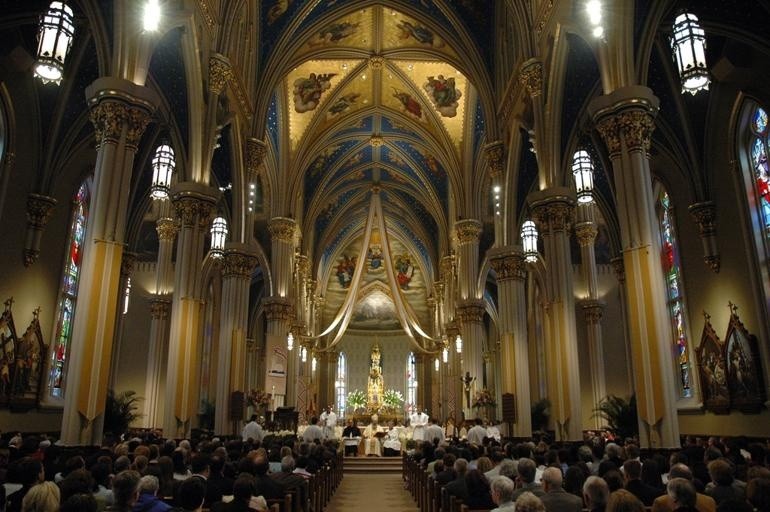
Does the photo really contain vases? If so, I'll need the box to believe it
[253,402,261,413]
[482,406,488,425]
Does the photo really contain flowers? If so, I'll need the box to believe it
[471,387,498,409]
[242,389,272,407]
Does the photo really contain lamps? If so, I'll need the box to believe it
[147,141,176,203]
[668,9,713,97]
[32,0,75,86]
[571,148,598,207]
[209,216,229,260]
[520,220,539,264]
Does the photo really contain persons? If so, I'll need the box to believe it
[1,428,336,512]
[383,421,401,457]
[405,427,770,512]
[298,73,321,105]
[363,413,384,457]
[427,74,456,107]
[242,405,336,442]
[410,405,500,443]
[342,419,361,455]
